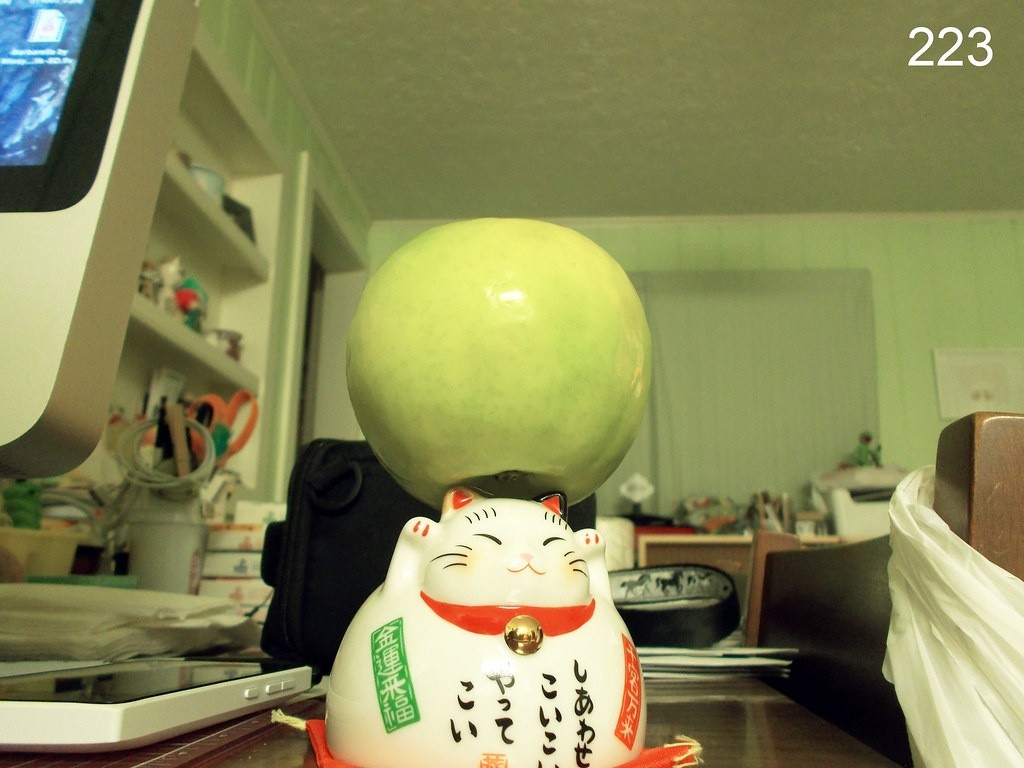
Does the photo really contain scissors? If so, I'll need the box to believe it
[188,387,259,480]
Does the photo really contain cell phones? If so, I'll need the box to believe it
[1,659,311,754]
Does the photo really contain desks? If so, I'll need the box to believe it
[1,696,317,768]
[185,673,899,768]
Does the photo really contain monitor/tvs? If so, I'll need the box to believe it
[1,1,202,481]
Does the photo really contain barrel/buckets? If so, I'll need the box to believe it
[125,506,208,599]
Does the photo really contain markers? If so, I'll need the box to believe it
[756,489,790,533]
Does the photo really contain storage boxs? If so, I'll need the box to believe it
[0,526,132,578]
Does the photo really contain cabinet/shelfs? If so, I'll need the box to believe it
[77,150,310,509]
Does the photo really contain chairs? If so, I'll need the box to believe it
[638,533,753,625]
[739,413,1024,766]
